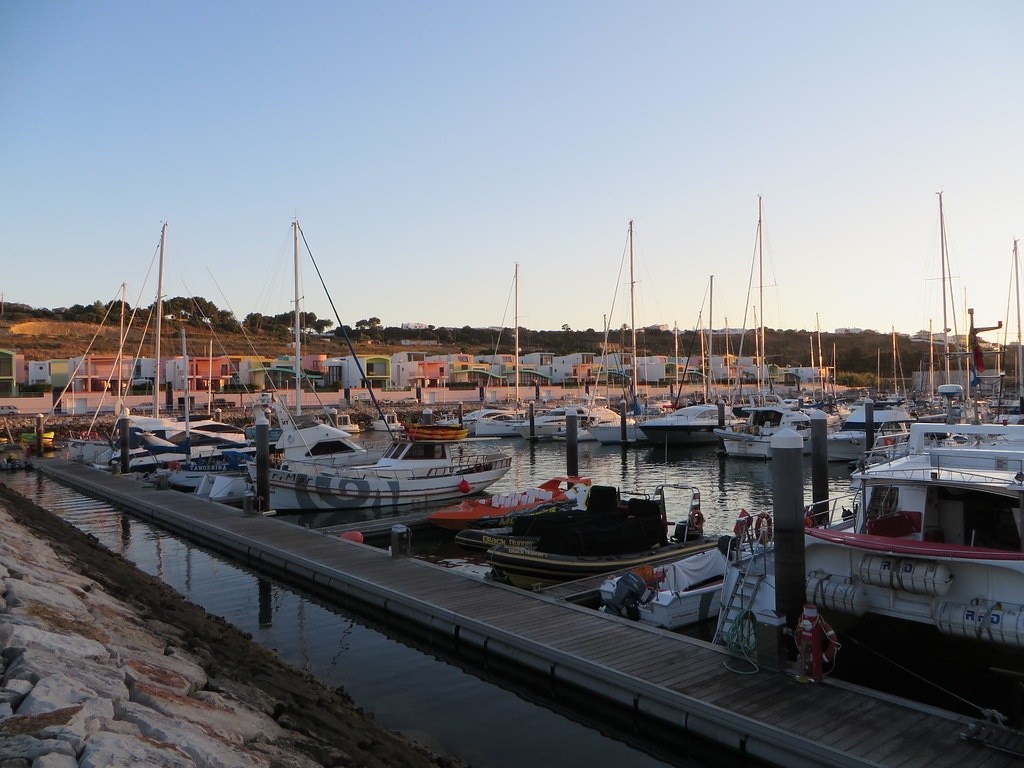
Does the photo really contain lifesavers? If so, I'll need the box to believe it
[794,613,839,664]
[756,512,774,546]
[690,509,704,528]
[884,436,893,448]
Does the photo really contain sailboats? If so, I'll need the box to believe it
[18,184,1024,694]
